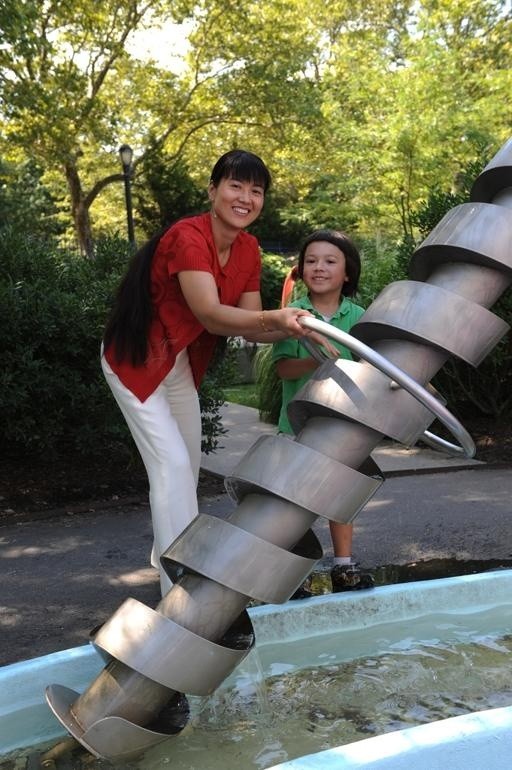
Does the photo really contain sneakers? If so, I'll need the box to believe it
[331,563,374,593]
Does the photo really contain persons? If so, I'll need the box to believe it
[273,229,376,600]
[98,149,342,601]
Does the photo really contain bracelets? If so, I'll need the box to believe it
[259,310,272,332]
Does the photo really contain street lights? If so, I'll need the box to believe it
[119,143,137,242]
